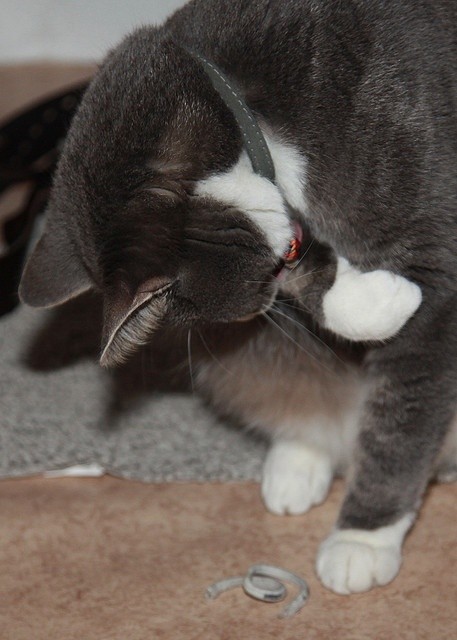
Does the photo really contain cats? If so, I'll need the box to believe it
[17,0,456,595]
